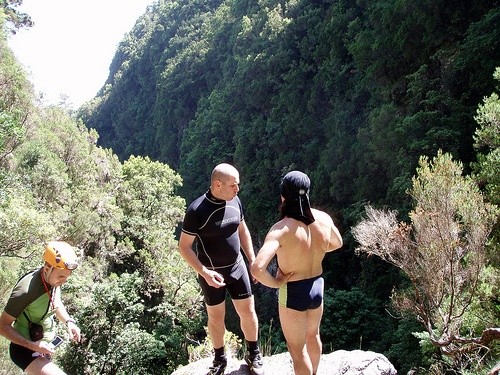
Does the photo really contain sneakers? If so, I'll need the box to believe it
[244,349,264,375]
[206,358,227,375]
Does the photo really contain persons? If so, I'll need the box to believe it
[178,162,263,375]
[0,241,82,374]
[250,170,343,374]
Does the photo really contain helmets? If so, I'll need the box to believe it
[42,241,78,270]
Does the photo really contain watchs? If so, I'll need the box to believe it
[63,317,75,326]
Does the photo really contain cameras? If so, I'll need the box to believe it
[48,336,63,349]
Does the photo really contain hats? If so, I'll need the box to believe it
[279,170,315,225]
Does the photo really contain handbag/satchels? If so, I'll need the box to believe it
[28,321,44,341]
